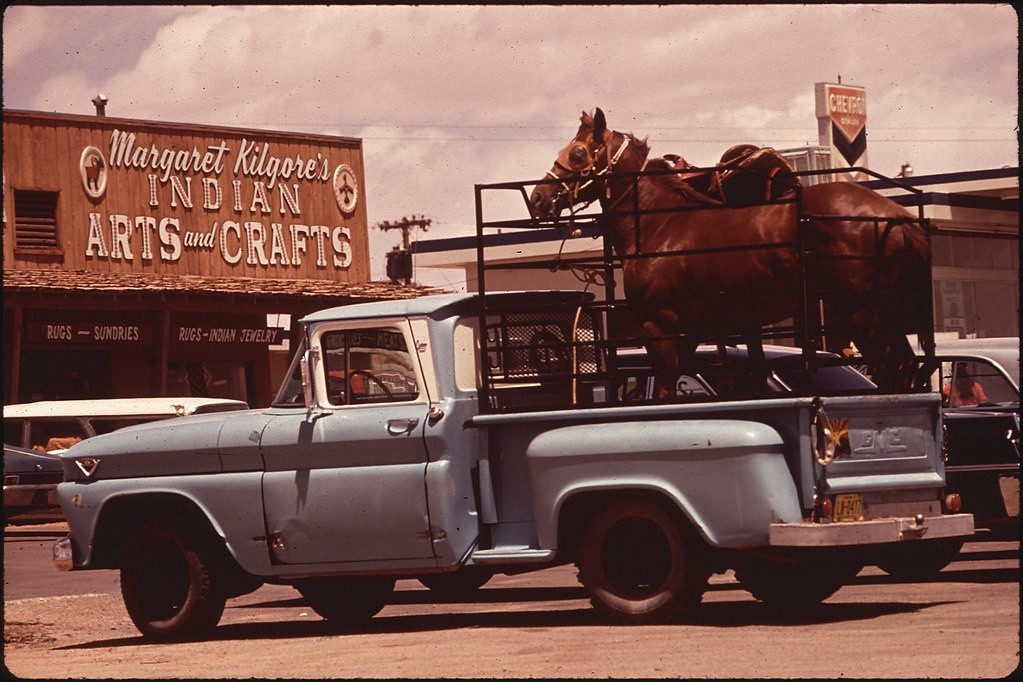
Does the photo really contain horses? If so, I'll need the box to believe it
[526,107,937,396]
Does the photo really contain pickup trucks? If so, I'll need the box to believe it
[47,157,974,636]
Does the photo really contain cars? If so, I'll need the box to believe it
[581,288,1019,586]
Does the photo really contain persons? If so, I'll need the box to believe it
[942,360,988,408]
